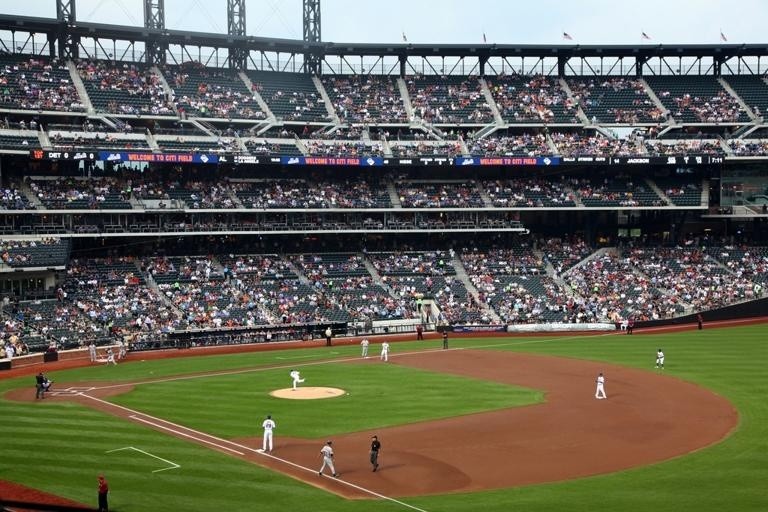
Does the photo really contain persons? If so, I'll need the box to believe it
[318,440,340,478]
[260,413,276,453]
[593,371,607,400]
[35,370,46,401]
[105,347,118,367]
[1,54,768,363]
[289,369,306,391]
[43,377,54,391]
[368,433,383,472]
[655,346,666,370]
[96,473,109,512]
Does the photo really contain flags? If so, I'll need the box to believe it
[564,33,572,40]
[403,33,407,42]
[720,31,726,41]
[641,32,649,40]
